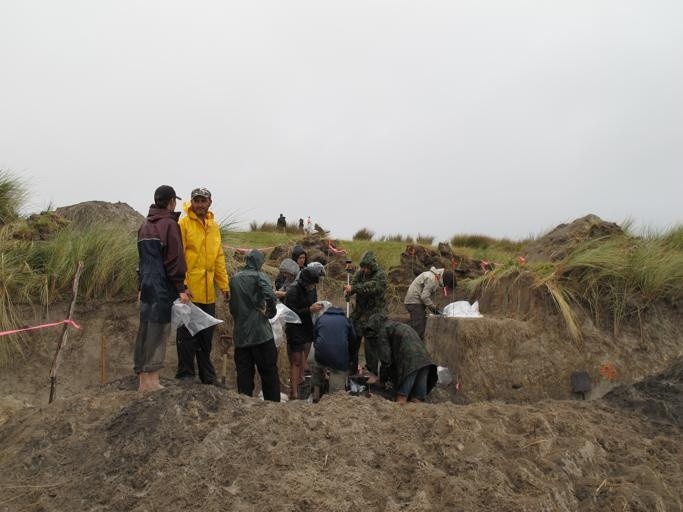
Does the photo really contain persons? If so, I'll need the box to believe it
[402,266,457,341]
[131,183,187,391]
[229,244,388,403]
[299,219,304,228]
[178,188,232,386]
[277,213,286,227]
[362,311,440,404]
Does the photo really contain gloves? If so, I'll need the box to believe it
[428,304,440,314]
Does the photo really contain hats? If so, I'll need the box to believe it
[191,188,211,199]
[154,185,182,200]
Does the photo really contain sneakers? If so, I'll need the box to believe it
[181,375,230,390]
